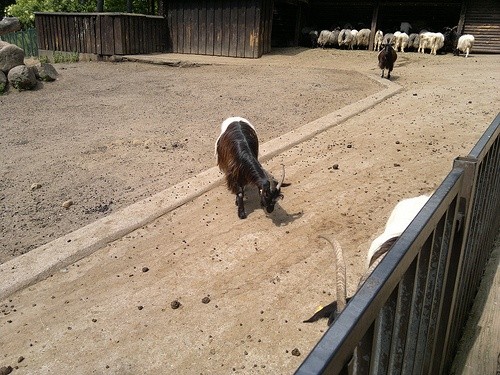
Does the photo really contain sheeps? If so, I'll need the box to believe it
[442,26,458,56]
[317,190,435,315]
[377,42,397,79]
[301,27,445,55]
[214,117,293,220]
[457,33,475,58]
